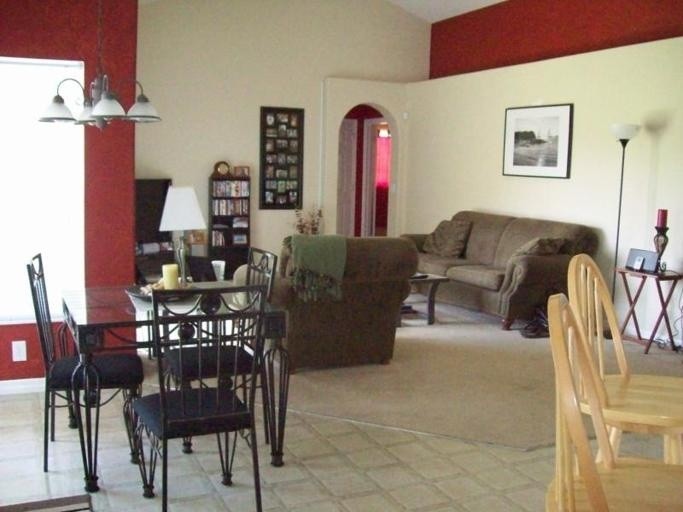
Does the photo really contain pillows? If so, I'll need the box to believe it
[419,219,473,261]
[511,235,564,257]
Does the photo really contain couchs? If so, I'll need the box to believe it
[399,208,599,332]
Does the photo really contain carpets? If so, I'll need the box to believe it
[133,304,682,453]
[0,492,92,511]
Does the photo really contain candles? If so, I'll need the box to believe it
[655,207,668,228]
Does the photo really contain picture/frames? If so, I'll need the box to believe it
[500,101,574,180]
[256,103,304,212]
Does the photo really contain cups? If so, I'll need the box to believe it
[162,264,178,291]
[211,260,227,288]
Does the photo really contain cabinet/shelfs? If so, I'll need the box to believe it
[208,175,251,278]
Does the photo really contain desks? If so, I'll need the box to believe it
[54,281,294,495]
[611,264,682,356]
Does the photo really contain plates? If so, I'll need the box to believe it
[124,284,195,301]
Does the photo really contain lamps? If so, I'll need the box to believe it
[157,184,207,287]
[608,120,642,304]
[37,0,162,131]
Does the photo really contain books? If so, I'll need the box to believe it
[211,180,249,247]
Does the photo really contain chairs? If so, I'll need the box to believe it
[163,244,279,454]
[544,289,682,512]
[24,251,144,476]
[232,234,420,377]
[561,250,682,465]
[126,280,273,512]
